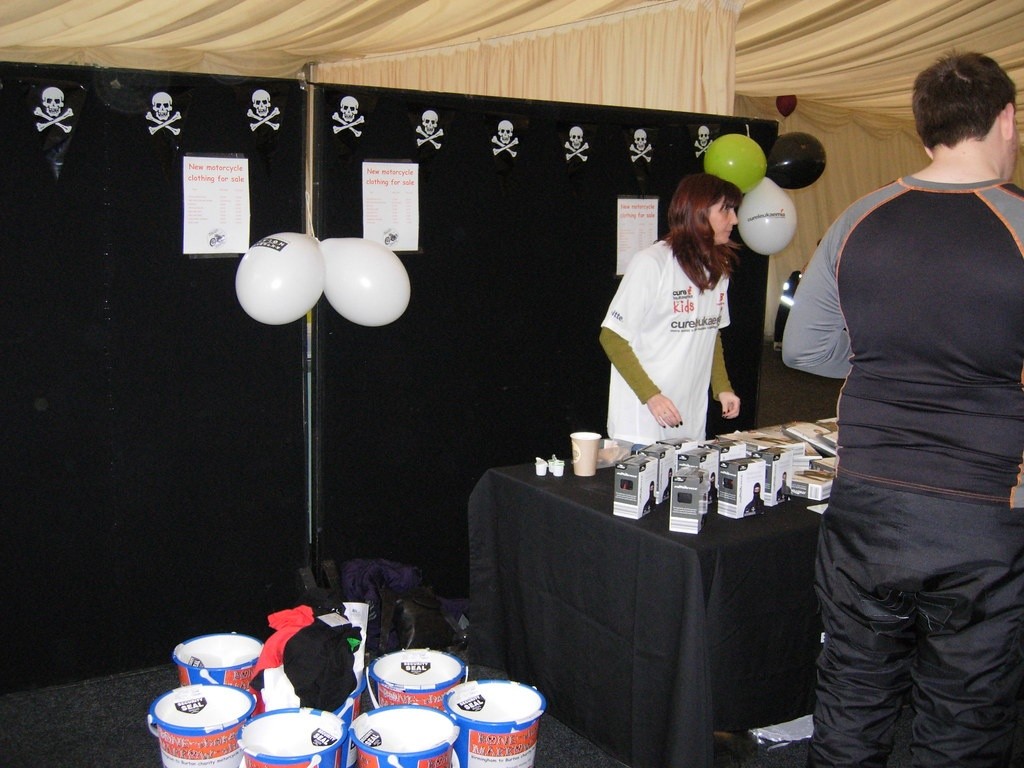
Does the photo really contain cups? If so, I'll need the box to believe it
[570,432,601,476]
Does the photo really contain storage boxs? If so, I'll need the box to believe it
[717,458,767,519]
[751,446,794,507]
[655,436,698,455]
[677,447,719,504]
[792,472,833,501]
[704,439,746,462]
[636,443,677,504]
[613,454,659,519]
[811,457,835,472]
[794,443,823,471]
[669,468,709,535]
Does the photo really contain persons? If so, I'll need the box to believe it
[600,171,743,445]
[783,50,1023,768]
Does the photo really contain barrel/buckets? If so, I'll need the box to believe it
[149,631,545,768]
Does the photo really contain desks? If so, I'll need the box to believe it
[467,419,829,768]
